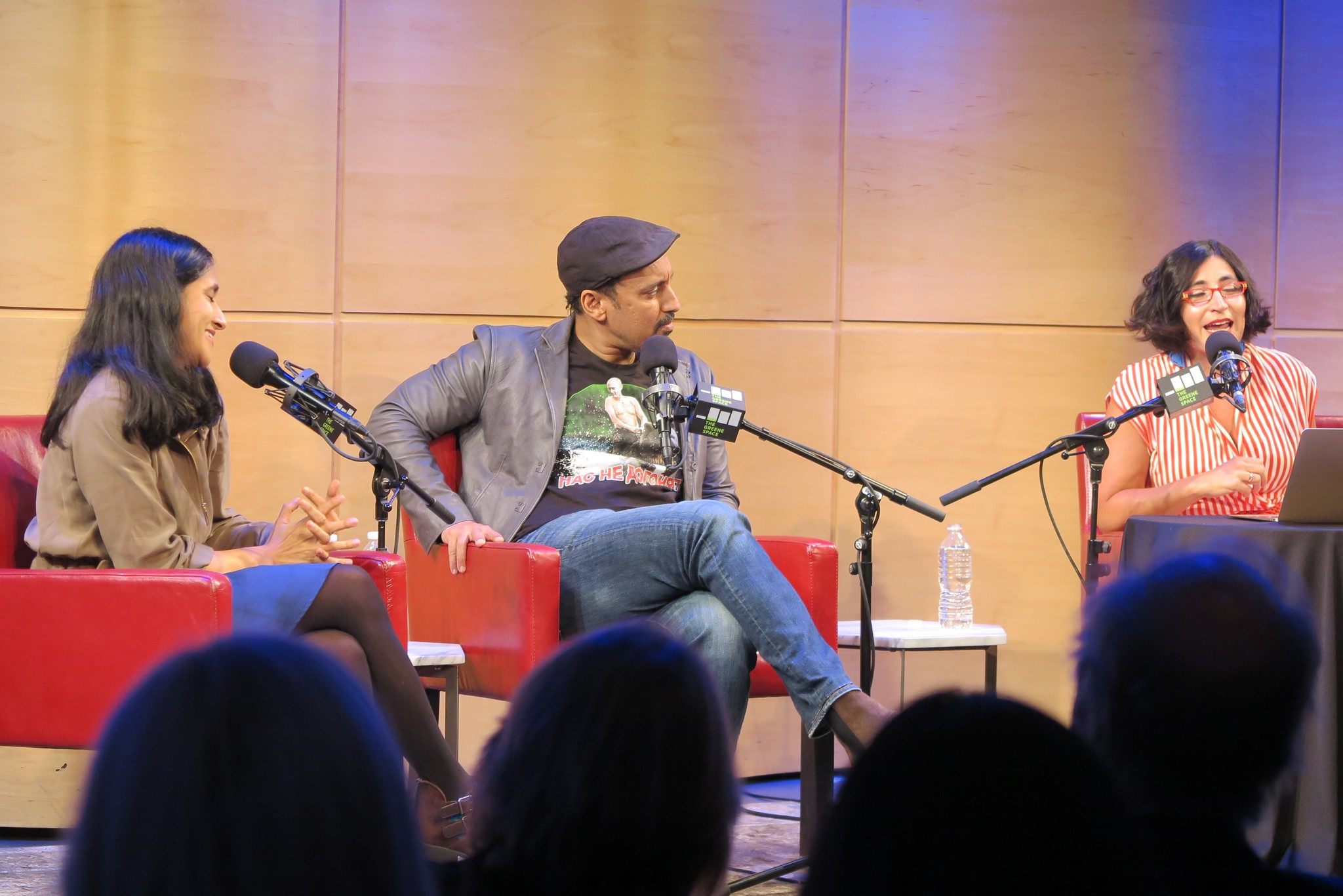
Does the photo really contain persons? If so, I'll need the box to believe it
[432,620,737,895]
[795,550,1343,896]
[62,632,423,895]
[368,217,896,769]
[24,228,479,865]
[604,376,650,454]
[1096,238,1319,535]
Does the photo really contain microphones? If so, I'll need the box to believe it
[1205,330,1248,413]
[228,341,372,436]
[640,334,678,466]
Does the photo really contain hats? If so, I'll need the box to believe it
[556,216,679,297]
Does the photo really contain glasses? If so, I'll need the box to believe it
[1179,282,1247,305]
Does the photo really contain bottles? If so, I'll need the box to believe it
[937,525,975,631]
[362,531,391,553]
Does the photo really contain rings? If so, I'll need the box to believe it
[1249,473,1254,482]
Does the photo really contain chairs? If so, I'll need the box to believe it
[0,416,838,856]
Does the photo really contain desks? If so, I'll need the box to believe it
[1118,515,1343,883]
[838,620,1007,711]
[408,641,465,761]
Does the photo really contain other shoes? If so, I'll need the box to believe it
[416,779,478,864]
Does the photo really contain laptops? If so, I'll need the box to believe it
[1230,427,1343,524]
